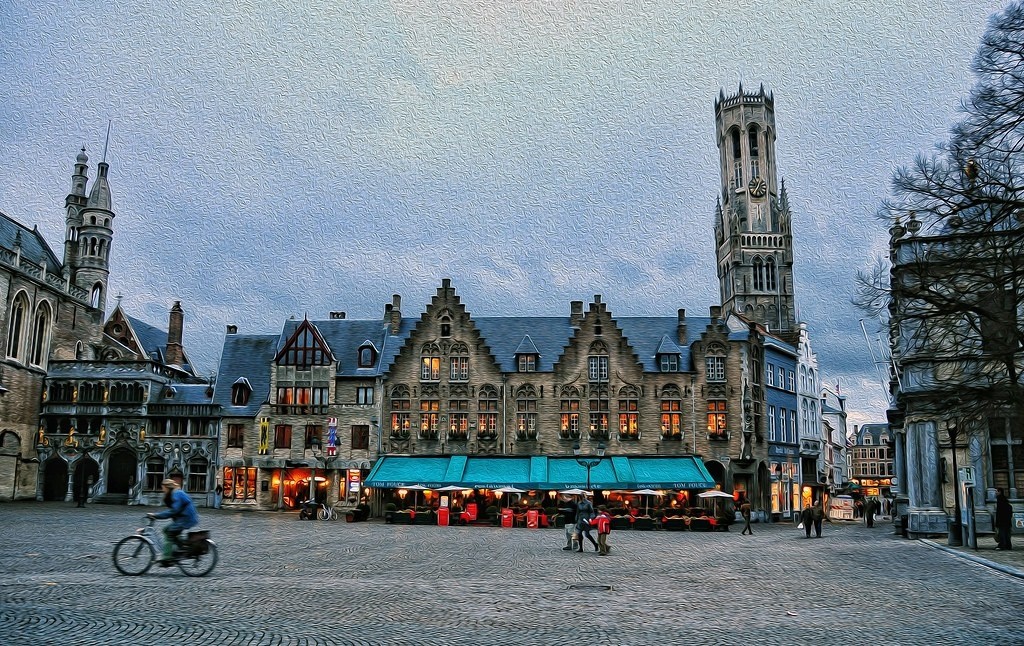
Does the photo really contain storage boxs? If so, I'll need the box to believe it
[187,530,211,542]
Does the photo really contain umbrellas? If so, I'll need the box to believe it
[631,488,665,514]
[435,484,470,509]
[492,486,526,505]
[559,489,593,496]
[697,491,734,516]
[402,484,428,510]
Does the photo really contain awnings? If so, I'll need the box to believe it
[363,456,715,490]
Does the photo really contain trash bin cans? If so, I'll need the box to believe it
[307,503,319,520]
[901,514,909,536]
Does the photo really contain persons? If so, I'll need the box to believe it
[147,478,198,563]
[800,500,824,538]
[575,491,599,552]
[994,487,1012,551]
[740,499,753,535]
[589,505,612,555]
[864,497,877,528]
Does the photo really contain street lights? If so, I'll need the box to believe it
[72,429,100,509]
[572,443,606,491]
[311,436,341,519]
[945,417,963,545]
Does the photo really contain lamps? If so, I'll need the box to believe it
[398,489,407,499]
[602,490,610,499]
[462,490,470,499]
[494,491,503,499]
[549,491,557,499]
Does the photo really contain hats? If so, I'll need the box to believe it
[598,505,606,511]
[162,479,178,488]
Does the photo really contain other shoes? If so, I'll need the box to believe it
[577,548,583,552]
[595,543,598,551]
[563,547,571,550]
[599,553,606,556]
[607,546,611,552]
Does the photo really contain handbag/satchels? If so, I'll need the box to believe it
[572,524,579,540]
[797,523,804,529]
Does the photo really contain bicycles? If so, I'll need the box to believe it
[317,503,338,521]
[111,516,218,577]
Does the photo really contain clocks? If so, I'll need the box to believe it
[749,177,766,197]
